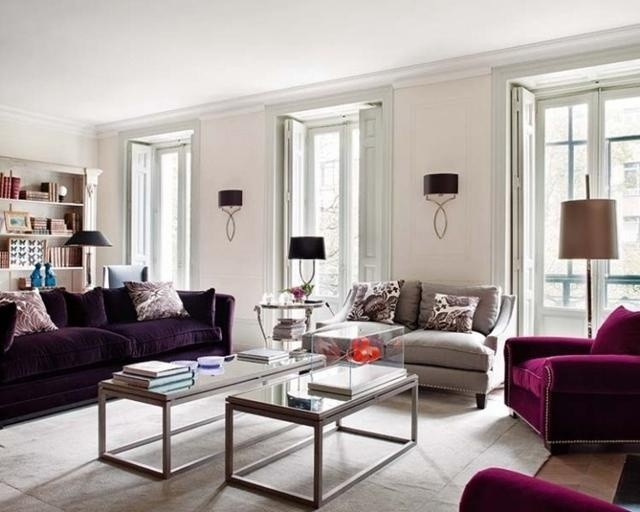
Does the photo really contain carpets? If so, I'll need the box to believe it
[0,386,551,512]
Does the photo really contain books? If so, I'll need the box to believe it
[111,358,198,395]
[0,168,83,268]
[236,348,289,363]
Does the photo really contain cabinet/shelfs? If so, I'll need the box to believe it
[253,298,326,342]
[0,155,104,294]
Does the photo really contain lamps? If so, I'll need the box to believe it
[558,173,622,339]
[217,189,243,242]
[288,236,327,300]
[423,173,459,241]
[63,231,115,292]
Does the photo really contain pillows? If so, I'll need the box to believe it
[347,278,404,326]
[0,288,60,337]
[395,282,422,329]
[424,292,481,334]
[122,279,191,321]
[417,281,501,333]
[62,287,109,326]
[186,288,216,329]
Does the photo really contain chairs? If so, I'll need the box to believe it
[102,263,149,289]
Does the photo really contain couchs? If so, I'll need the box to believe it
[0,286,236,426]
[301,278,517,411]
[501,303,640,456]
[459,467,640,512]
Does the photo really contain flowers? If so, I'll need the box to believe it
[279,281,315,304]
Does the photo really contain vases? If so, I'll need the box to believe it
[291,295,307,304]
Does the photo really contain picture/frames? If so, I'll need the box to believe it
[4,211,33,231]
[8,237,49,268]
